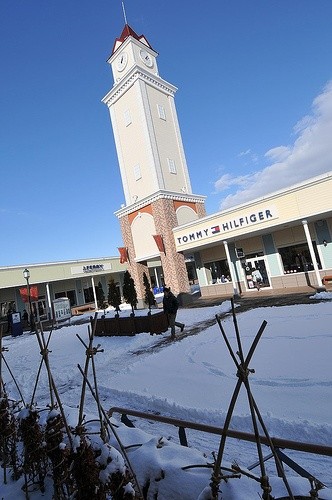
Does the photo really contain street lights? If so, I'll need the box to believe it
[23,268,39,335]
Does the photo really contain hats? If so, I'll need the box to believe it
[163,286,171,294]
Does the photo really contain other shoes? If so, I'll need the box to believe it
[170,334,176,340]
[180,324,186,333]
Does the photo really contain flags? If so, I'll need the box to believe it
[117,246,128,265]
[152,234,165,253]
[29,285,39,302]
[19,286,29,303]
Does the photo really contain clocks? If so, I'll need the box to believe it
[139,48,153,68]
[116,51,128,72]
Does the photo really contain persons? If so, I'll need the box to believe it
[294,249,309,271]
[250,266,264,292]
[7,310,15,333]
[212,274,231,284]
[6,306,14,330]
[21,308,30,328]
[162,286,186,340]
